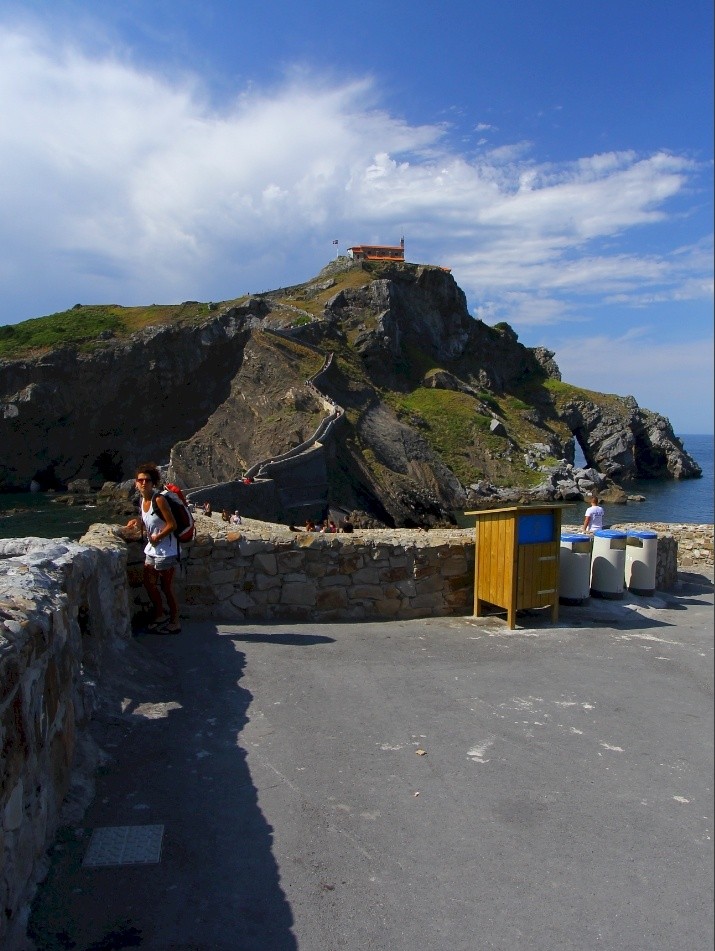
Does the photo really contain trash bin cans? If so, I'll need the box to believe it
[590,530,627,601]
[625,530,658,597]
[464,503,576,630]
[560,533,593,607]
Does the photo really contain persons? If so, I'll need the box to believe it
[185,497,355,535]
[581,496,605,533]
[124,464,183,636]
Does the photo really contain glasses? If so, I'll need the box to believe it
[136,478,151,484]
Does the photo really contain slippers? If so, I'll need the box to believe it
[157,623,182,635]
[146,619,170,631]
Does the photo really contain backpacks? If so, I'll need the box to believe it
[151,483,196,544]
